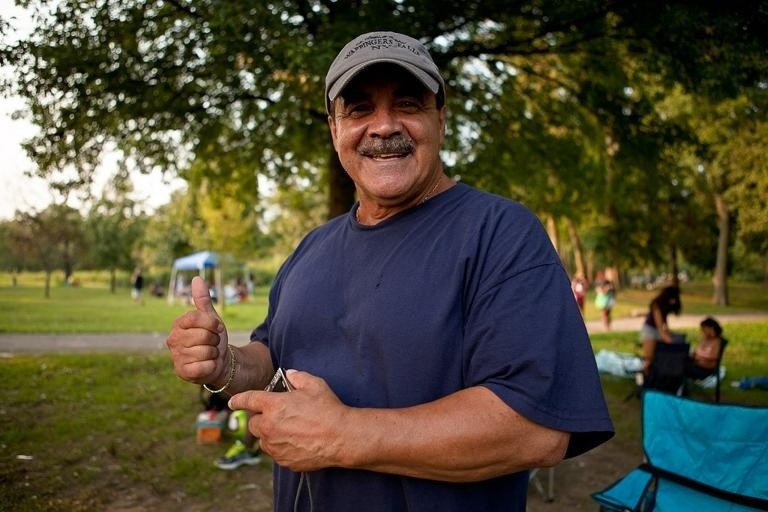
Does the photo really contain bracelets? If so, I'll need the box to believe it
[202,342,237,394]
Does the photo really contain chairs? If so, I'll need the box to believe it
[619,331,733,405]
[588,387,767,512]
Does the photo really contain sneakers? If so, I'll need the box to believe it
[216,440,261,470]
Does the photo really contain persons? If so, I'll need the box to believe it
[640,286,681,374]
[129,266,256,304]
[165,30,617,511]
[654,316,721,392]
[200,383,264,470]
[571,268,692,331]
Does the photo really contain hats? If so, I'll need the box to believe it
[324,30,447,116]
